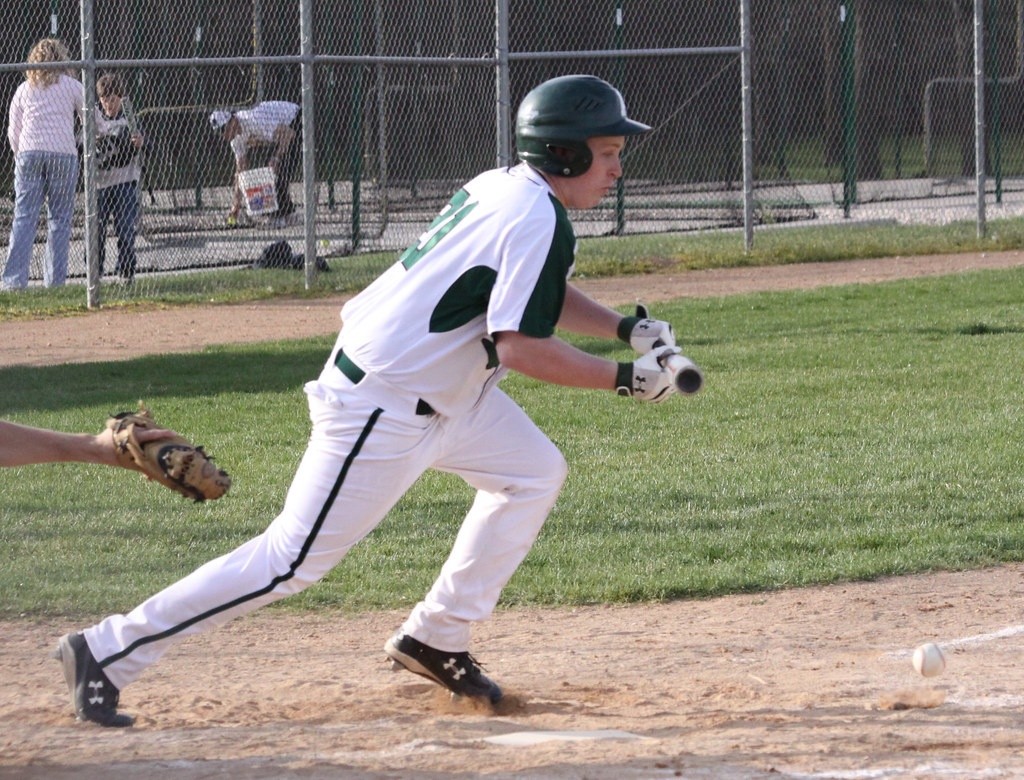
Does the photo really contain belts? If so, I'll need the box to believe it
[335,350,435,417]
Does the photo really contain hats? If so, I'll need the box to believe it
[209,111,231,131]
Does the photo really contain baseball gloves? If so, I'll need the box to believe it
[109,399,233,504]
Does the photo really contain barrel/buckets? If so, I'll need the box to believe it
[238,166,280,218]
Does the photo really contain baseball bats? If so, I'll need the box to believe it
[652,340,706,397]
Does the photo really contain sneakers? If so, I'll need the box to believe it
[51,631,136,728]
[384,630,502,703]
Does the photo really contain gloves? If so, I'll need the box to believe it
[615,345,686,403]
[617,315,677,354]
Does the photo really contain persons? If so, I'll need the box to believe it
[209,100,304,229]
[0,39,108,292]
[50,73,683,726]
[0,410,234,504]
[94,73,145,287]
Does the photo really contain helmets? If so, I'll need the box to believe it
[515,74,652,176]
